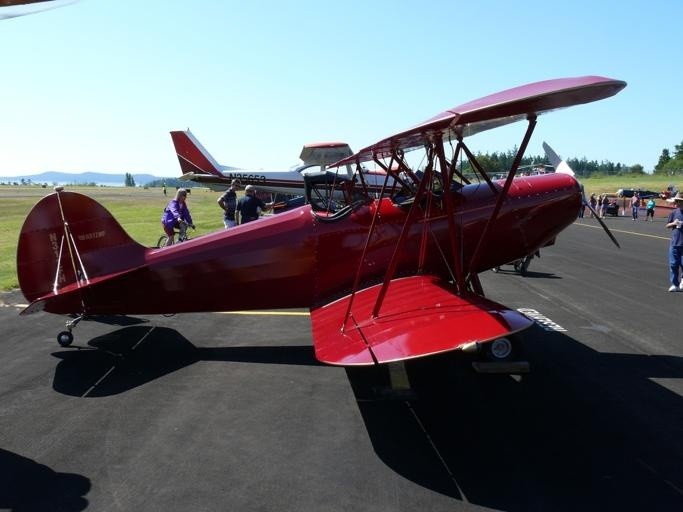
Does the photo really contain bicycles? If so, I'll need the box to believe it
[157,219,196,249]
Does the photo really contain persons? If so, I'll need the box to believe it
[217,179,242,230]
[162,182,167,196]
[665,193,683,292]
[160,187,196,247]
[235,185,274,227]
[578,190,672,223]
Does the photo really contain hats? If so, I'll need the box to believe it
[666,192,683,202]
[245,185,255,191]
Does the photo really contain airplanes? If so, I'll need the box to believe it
[15,73,627,369]
[615,185,678,199]
[167,129,435,208]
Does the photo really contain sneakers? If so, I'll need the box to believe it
[668,278,683,292]
